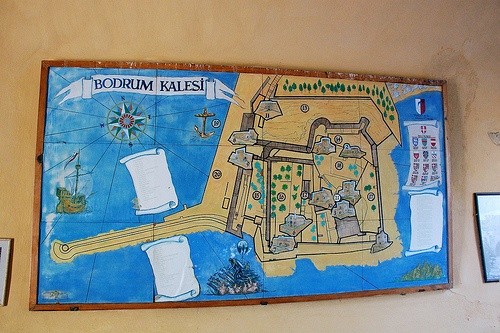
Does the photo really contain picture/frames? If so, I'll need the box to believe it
[0,237,15,307]
[28,60,454,311]
[474,191,500,283]
